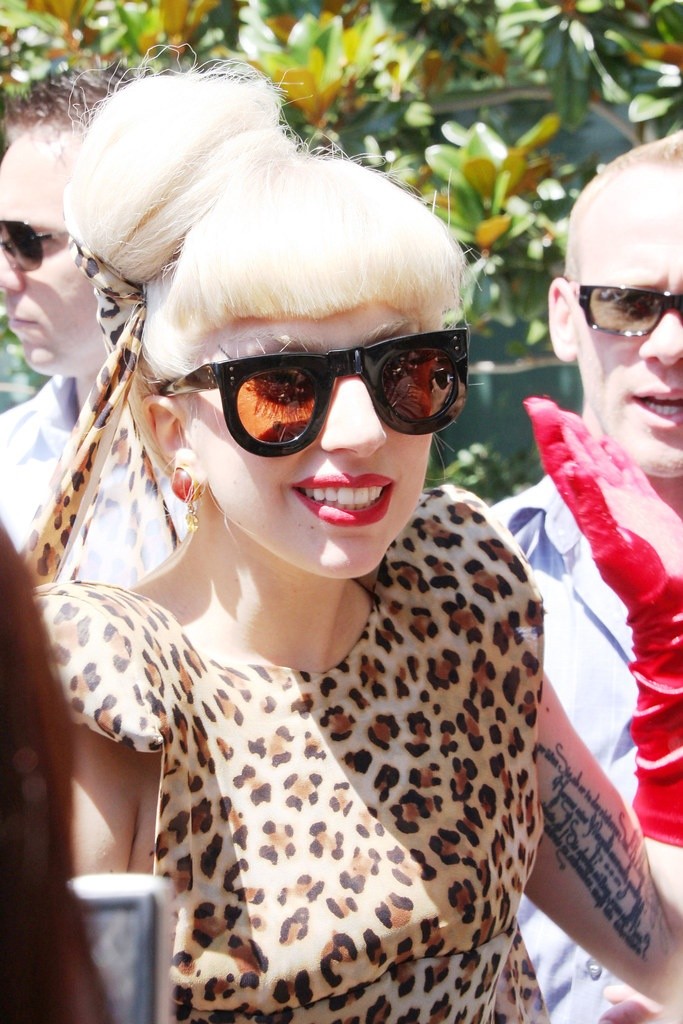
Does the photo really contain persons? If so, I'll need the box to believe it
[0,68,683,1024]
[0,62,193,590]
[0,524,118,1024]
[488,128,683,1023]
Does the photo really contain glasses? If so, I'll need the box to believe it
[577,284,683,337]
[0,220,53,272]
[159,328,471,458]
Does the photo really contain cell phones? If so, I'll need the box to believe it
[71,873,176,1024]
